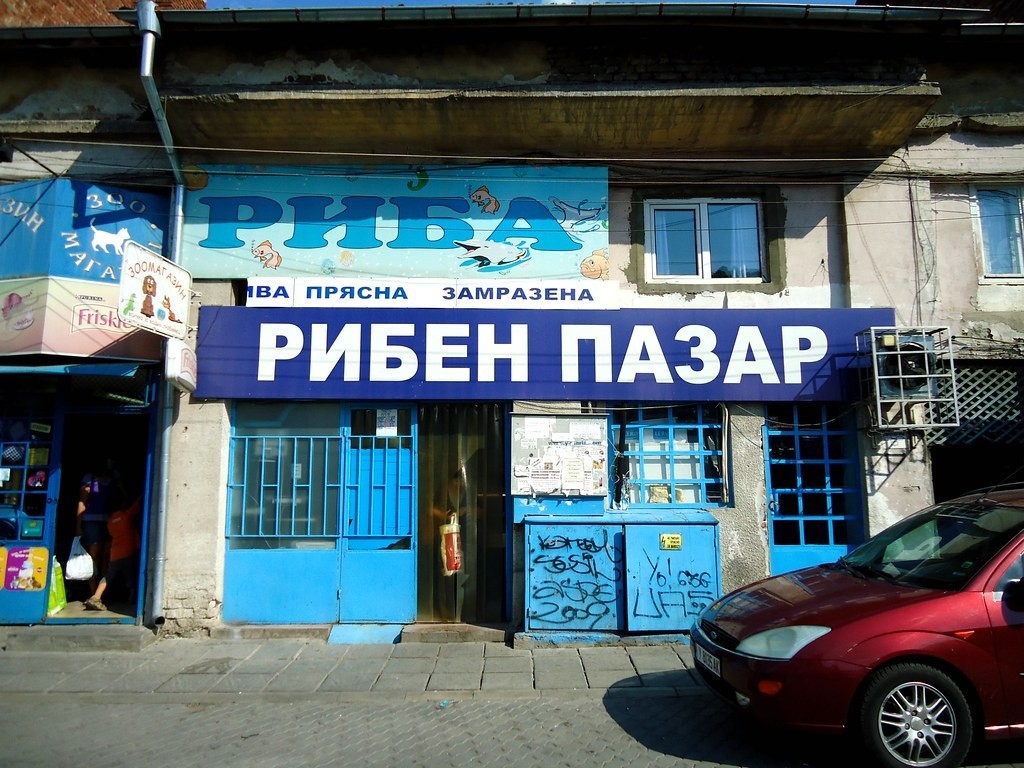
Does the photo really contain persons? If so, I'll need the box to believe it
[74,459,145,611]
[428,471,483,623]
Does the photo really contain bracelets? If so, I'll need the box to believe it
[76,528,82,531]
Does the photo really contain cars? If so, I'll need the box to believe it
[687,484,1024,768]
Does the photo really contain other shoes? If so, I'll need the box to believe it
[87,594,108,611]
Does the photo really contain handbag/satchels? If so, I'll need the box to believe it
[63,535,98,581]
[438,512,466,577]
